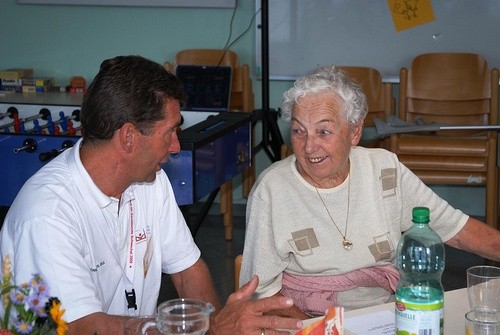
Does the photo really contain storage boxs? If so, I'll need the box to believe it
[0,68,53,96]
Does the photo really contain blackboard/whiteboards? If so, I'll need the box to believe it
[254,0,500,83]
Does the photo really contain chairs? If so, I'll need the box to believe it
[157,48,259,240]
[277,51,499,269]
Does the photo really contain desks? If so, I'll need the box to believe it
[0,87,86,106]
[270,280,500,334]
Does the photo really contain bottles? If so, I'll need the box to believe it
[395,207,445,335]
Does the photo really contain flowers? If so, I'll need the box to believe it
[1,252,71,334]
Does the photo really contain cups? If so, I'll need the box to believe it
[465,265,500,322]
[465,311,500,335]
[140,299,215,335]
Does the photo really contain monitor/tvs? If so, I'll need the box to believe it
[176,63,233,110]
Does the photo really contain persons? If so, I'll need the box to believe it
[239,68,500,321]
[0,56,302,335]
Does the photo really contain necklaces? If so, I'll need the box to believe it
[312,173,352,250]
